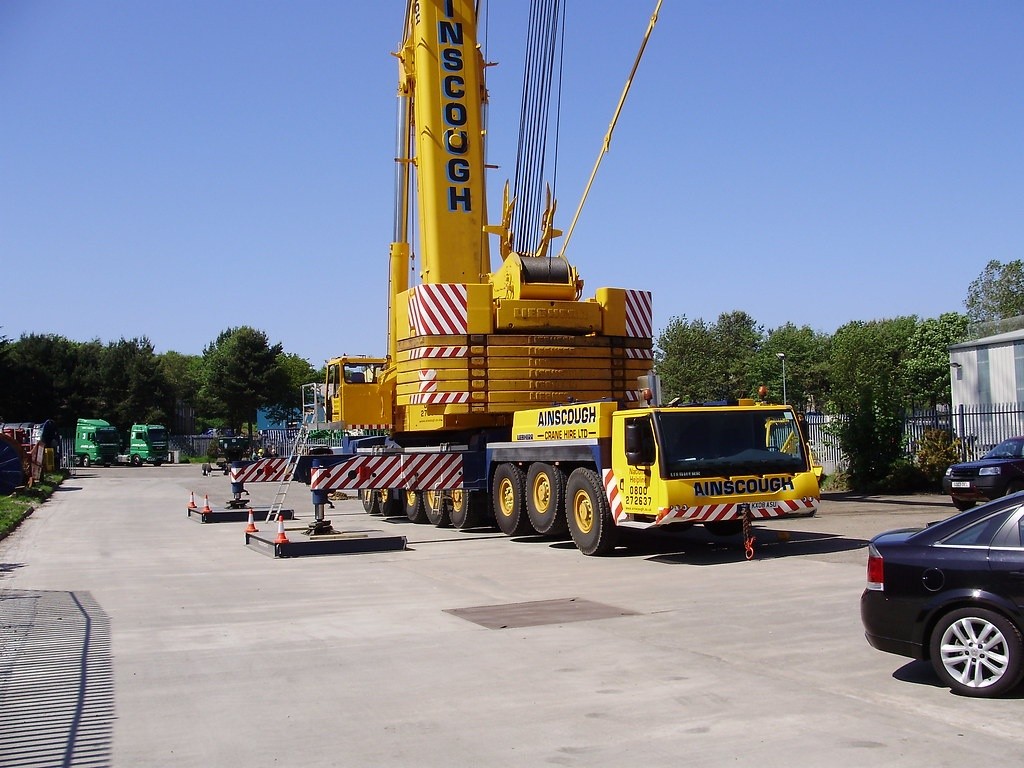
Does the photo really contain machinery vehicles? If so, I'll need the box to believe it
[314,1,825,557]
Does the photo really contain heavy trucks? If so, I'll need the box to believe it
[113,424,171,467]
[0,417,121,468]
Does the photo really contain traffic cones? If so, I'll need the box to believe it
[244,507,260,533]
[274,515,291,543]
[203,495,214,512]
[188,491,198,509]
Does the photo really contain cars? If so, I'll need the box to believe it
[859,487,1024,698]
[941,436,1024,512]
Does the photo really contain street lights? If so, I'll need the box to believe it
[775,352,787,405]
